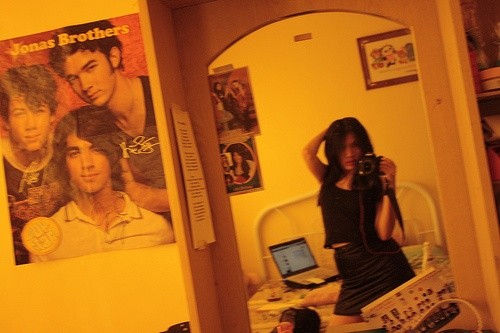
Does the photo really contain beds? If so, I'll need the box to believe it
[246,179,444,333]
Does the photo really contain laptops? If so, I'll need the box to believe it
[268,237,341,288]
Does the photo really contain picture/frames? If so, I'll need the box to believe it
[357,28,419,90]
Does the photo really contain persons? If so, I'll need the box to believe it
[0,63,72,264]
[381,289,457,333]
[302,117,417,327]
[232,148,249,178]
[29,104,174,264]
[215,80,251,131]
[50,20,173,222]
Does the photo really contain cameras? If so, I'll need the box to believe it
[358,153,385,176]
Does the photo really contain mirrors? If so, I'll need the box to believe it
[207,10,458,333]
